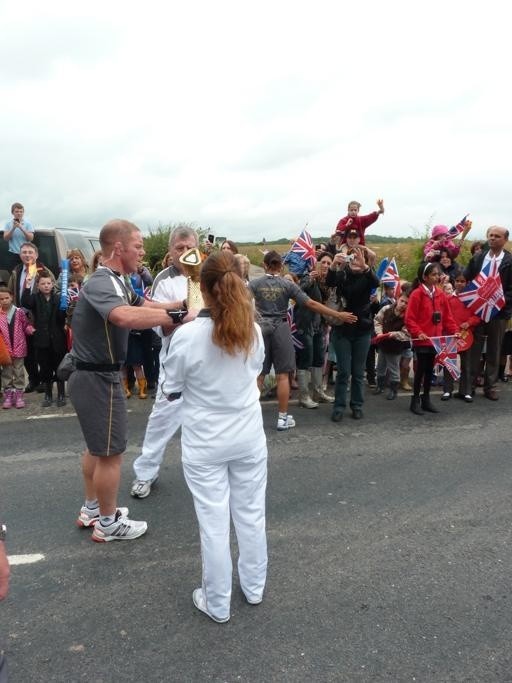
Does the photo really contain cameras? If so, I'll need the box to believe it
[344,255,355,262]
[433,312,441,324]
[434,249,440,255]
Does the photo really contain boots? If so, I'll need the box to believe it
[122,378,131,399]
[410,393,440,415]
[367,379,399,400]
[137,377,148,399]
[2,380,65,409]
[400,365,413,391]
[299,384,335,409]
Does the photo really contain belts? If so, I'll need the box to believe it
[72,357,120,372]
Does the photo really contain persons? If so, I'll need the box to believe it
[3,203,35,277]
[159,242,271,622]
[246,201,512,430]
[0,242,102,411]
[122,251,173,399]
[130,225,205,501]
[0,520,19,681]
[70,216,199,542]
[201,238,250,284]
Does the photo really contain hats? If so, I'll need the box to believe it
[345,227,361,236]
[429,224,448,239]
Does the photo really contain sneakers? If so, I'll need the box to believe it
[441,388,498,402]
[276,415,295,431]
[193,588,230,623]
[130,473,159,499]
[331,411,343,422]
[76,505,149,542]
[352,409,363,420]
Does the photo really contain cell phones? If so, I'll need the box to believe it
[317,261,321,268]
[14,219,20,223]
[316,245,321,250]
[208,234,214,243]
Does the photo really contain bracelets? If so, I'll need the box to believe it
[0,523,7,539]
